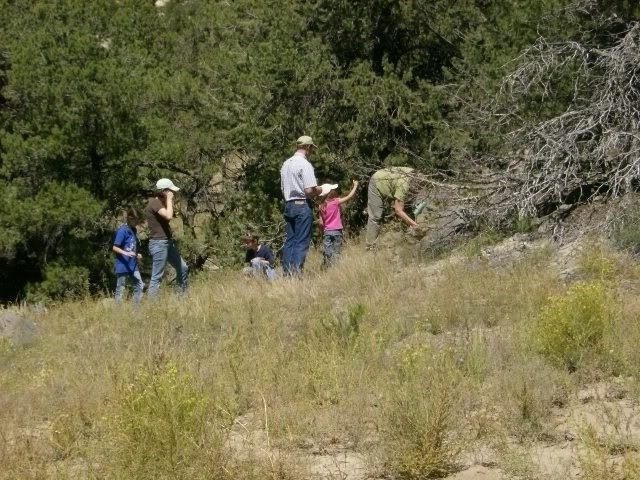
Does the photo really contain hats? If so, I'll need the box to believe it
[156,178,180,192]
[296,136,319,149]
[318,184,338,196]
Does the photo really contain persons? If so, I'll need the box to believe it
[111,207,144,306]
[314,180,359,267]
[140,178,190,306]
[240,234,278,282]
[366,166,434,251]
[277,135,322,276]
